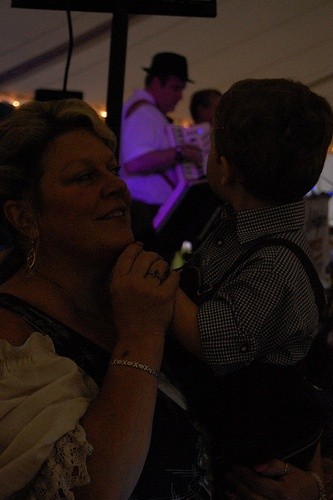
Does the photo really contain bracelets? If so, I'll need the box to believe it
[306,471,325,500]
[107,359,161,379]
[174,144,184,164]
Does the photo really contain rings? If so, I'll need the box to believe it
[147,269,164,280]
[279,462,288,475]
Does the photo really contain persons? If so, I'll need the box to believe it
[118,51,203,266]
[0,98,333,500]
[188,88,222,124]
[167,77,333,500]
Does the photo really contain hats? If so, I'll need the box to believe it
[142,51,196,84]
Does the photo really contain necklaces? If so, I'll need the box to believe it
[34,270,115,332]
